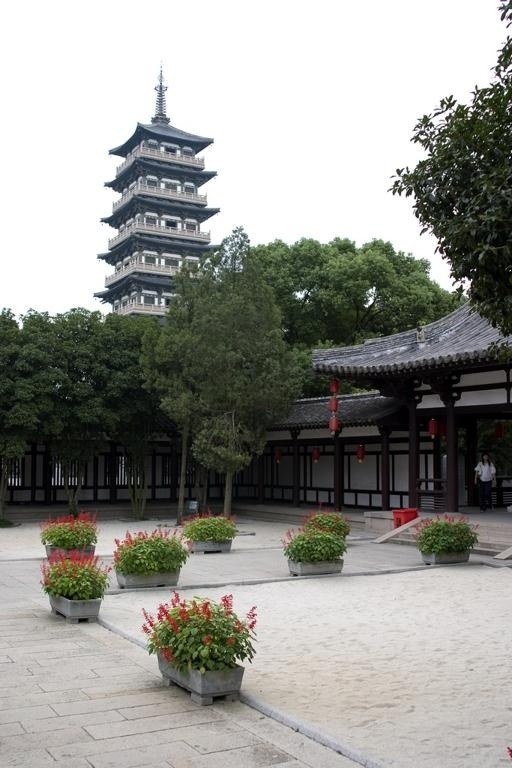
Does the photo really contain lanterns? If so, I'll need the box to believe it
[329,416,339,436]
[330,377,340,395]
[330,398,339,414]
[311,447,320,463]
[428,418,439,440]
[274,447,281,464]
[495,421,505,441]
[440,422,446,440]
[357,444,365,463]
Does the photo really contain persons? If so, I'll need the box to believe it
[474,452,497,512]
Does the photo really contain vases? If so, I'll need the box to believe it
[46,593,103,623]
[156,650,244,705]
[189,539,232,554]
[419,548,471,565]
[115,570,180,588]
[287,558,344,576]
[45,544,96,559]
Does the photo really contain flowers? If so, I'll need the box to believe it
[111,526,190,576]
[39,506,98,546]
[181,507,239,543]
[139,586,258,674]
[39,543,113,601]
[283,526,348,561]
[413,512,479,552]
[301,502,352,538]
